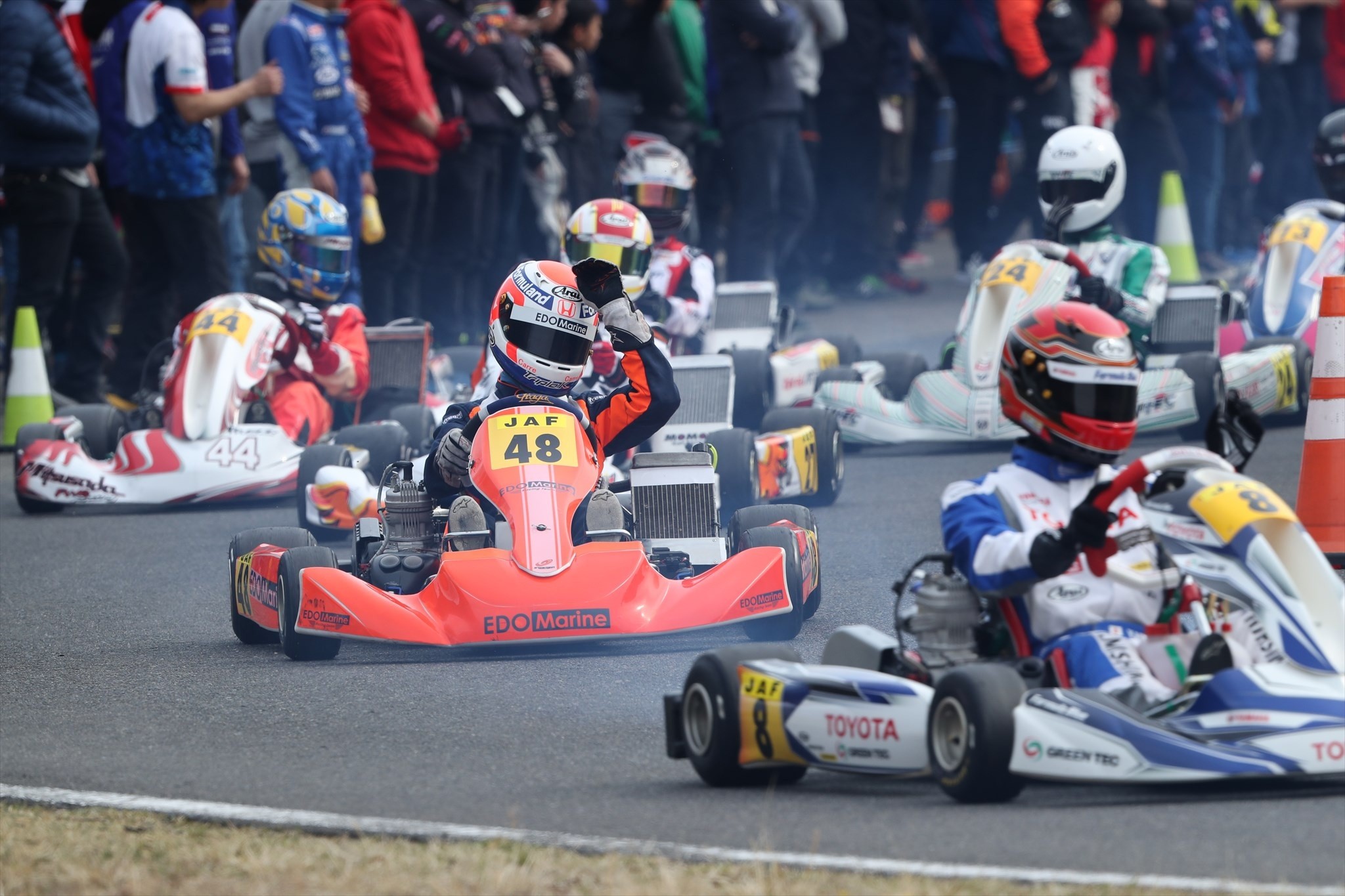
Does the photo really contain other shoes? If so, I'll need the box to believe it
[449,493,486,552]
[586,488,625,542]
[1184,634,1234,694]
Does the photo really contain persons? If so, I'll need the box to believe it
[132,187,369,443]
[0,0,1345,402]
[942,303,1286,716]
[563,139,716,465]
[422,257,682,539]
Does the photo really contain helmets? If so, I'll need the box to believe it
[999,303,1139,464]
[1315,110,1345,191]
[255,186,352,301]
[561,198,653,302]
[490,261,598,397]
[613,141,695,238]
[1038,122,1126,236]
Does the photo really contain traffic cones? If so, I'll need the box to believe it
[1152,171,1204,286]
[1292,275,1344,572]
[0,306,56,452]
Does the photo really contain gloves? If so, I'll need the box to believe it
[289,301,326,345]
[1077,277,1111,308]
[435,428,471,475]
[1057,481,1118,561]
[571,257,627,308]
[1041,195,1074,242]
